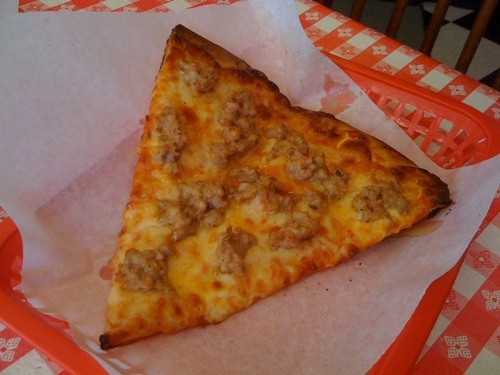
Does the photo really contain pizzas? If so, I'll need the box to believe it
[99,23,453,351]
